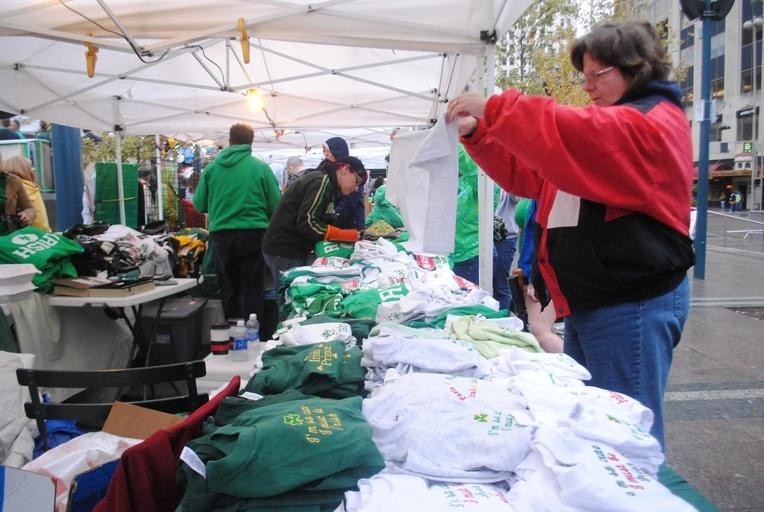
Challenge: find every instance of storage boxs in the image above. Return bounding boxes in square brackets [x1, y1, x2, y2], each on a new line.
[0, 402, 186, 511]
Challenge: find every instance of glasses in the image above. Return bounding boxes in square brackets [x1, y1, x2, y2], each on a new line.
[337, 163, 361, 185]
[583, 65, 615, 81]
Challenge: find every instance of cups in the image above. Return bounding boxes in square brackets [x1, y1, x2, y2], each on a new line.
[210, 316, 245, 354]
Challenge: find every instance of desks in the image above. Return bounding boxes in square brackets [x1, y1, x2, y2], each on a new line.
[47, 275, 199, 400]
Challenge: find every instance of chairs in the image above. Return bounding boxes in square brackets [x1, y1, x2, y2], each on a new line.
[14, 358, 210, 454]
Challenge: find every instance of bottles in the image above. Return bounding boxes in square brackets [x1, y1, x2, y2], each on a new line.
[236, 314, 260, 360]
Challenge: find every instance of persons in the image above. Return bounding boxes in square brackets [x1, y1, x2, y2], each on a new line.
[443, 21, 694, 461]
[140, 168, 208, 231]
[192, 123, 386, 344]
[0, 118, 50, 238]
[728, 192, 736, 213]
[450, 142, 566, 352]
[720, 191, 726, 211]
